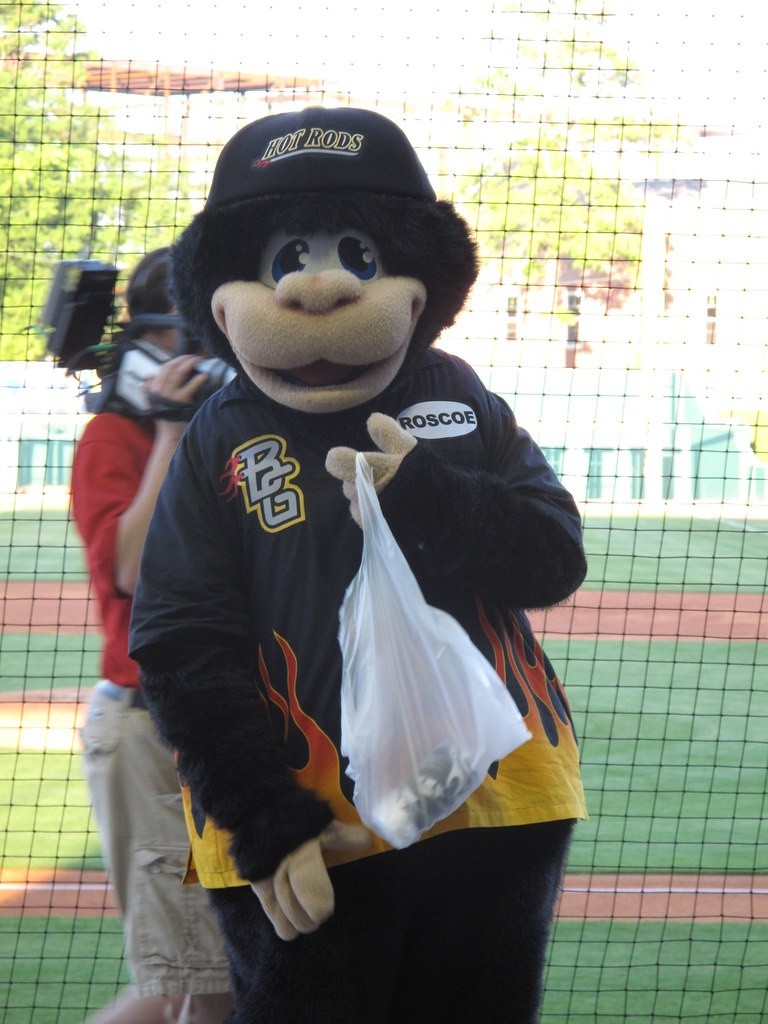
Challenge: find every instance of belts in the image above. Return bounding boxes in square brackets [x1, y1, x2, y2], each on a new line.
[95, 678, 146, 710]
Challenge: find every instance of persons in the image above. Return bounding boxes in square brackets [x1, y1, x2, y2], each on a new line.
[71, 248, 234, 1022]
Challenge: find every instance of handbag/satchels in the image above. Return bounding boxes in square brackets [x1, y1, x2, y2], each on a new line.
[335, 450, 533, 850]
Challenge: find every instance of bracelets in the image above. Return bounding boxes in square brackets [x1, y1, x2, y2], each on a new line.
[147, 393, 193, 423]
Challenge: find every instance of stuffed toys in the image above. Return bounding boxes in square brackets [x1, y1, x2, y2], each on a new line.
[125, 107, 591, 1024]
